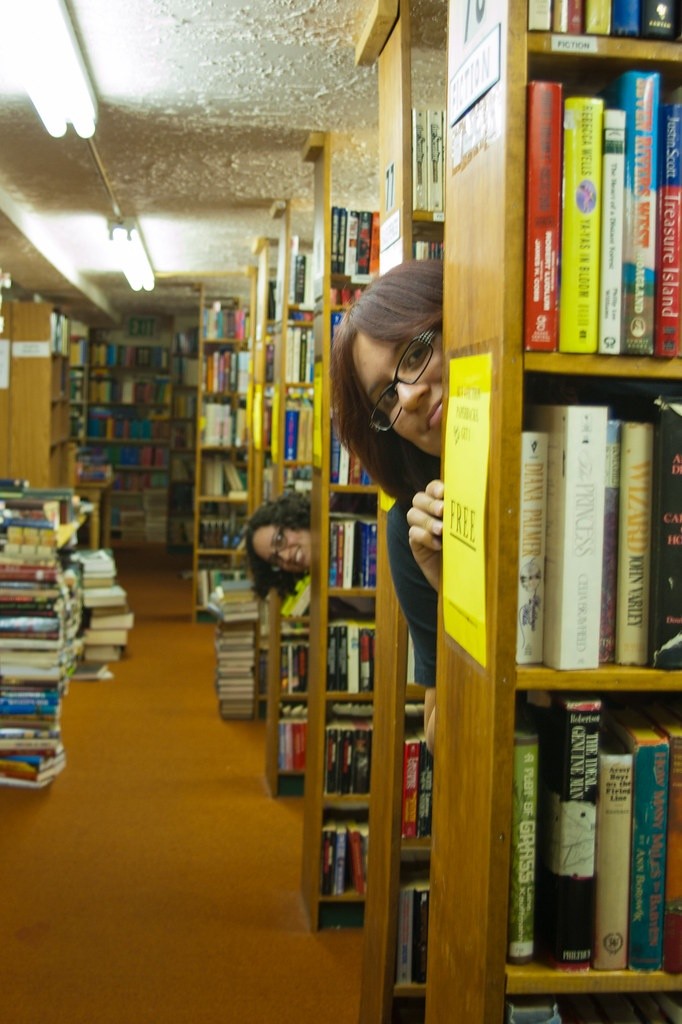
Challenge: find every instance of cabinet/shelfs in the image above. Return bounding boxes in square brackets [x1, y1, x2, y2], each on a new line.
[0, 0, 682, 1024]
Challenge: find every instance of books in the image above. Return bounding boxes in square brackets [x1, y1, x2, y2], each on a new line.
[0, 0, 682, 1024]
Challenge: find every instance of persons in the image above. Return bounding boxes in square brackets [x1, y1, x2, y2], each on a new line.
[328, 255, 444, 753]
[246, 491, 314, 597]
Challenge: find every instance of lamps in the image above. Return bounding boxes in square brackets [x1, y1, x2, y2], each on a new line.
[107, 217, 155, 291]
[0, 0, 99, 139]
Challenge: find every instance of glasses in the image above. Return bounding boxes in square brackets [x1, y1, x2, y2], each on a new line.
[368, 330, 436, 432]
[268, 526, 288, 573]
